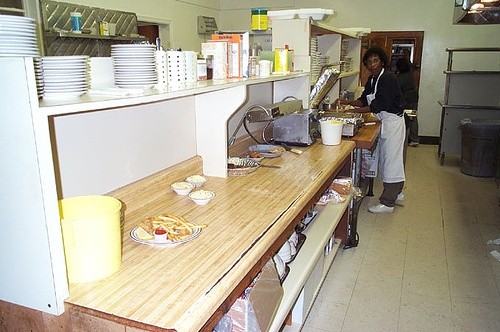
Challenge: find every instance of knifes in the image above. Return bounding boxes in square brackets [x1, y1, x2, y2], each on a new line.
[260, 164, 281, 168]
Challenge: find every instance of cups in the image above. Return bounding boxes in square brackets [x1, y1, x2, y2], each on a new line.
[259, 60, 274, 76]
[69, 12, 82, 34]
[251, 8, 269, 32]
[153, 229, 168, 241]
[108, 23, 117, 35]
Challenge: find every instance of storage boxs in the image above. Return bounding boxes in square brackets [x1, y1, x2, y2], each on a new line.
[226, 258, 284, 332]
[353, 136, 380, 177]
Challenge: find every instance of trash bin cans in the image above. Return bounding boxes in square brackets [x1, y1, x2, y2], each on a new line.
[458, 117, 500, 178]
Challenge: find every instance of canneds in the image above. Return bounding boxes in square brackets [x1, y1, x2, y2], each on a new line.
[250, 8, 268, 31]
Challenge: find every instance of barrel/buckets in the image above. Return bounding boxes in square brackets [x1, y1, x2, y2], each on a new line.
[58, 194, 122, 289]
[318, 117, 346, 146]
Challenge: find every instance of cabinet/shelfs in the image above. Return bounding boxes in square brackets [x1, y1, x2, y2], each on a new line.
[0, 16, 383, 332]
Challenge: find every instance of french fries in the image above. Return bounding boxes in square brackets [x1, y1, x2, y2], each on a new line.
[140, 214, 208, 242]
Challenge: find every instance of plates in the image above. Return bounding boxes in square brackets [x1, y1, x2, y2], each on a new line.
[129, 221, 201, 248]
[239, 152, 264, 161]
[249, 144, 283, 158]
[228, 161, 261, 177]
[309, 37, 329, 81]
[339, 39, 354, 73]
[0, 15, 158, 101]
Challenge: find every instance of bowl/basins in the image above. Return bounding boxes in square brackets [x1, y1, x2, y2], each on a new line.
[184, 175, 208, 188]
[271, 228, 298, 281]
[323, 104, 336, 111]
[170, 181, 195, 196]
[189, 190, 215, 205]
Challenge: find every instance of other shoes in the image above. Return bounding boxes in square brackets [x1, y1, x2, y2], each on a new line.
[368, 203, 395, 214]
[394, 194, 405, 207]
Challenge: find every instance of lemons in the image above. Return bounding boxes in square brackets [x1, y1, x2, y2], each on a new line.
[137, 227, 153, 240]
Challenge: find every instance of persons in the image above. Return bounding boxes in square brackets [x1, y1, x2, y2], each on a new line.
[395, 57, 419, 146]
[338, 46, 411, 213]
